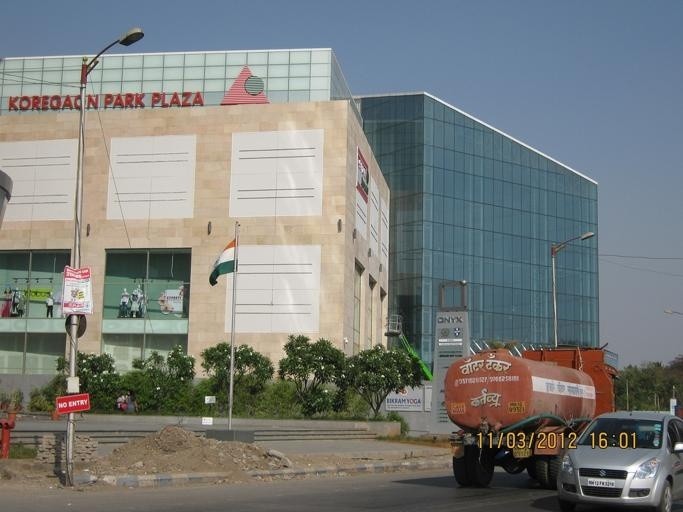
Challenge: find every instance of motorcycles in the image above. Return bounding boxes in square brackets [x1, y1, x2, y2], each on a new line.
[117, 295, 145, 319]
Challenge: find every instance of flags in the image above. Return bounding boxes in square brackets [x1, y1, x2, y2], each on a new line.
[209, 235, 235, 287]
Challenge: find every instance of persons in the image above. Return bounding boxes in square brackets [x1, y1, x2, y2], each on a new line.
[1, 284, 25, 317]
[125, 390, 139, 413]
[45, 291, 55, 318]
[119, 284, 148, 318]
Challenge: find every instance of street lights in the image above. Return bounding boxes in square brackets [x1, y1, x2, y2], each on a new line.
[65, 27, 145, 489]
[550, 231, 595, 348]
[664, 308, 683, 316]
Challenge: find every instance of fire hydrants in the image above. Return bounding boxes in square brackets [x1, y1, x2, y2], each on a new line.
[0, 411, 17, 461]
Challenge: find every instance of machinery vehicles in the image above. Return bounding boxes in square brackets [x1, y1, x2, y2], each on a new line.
[385, 314, 434, 382]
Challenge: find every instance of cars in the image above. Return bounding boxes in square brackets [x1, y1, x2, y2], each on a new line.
[555, 412, 682, 512]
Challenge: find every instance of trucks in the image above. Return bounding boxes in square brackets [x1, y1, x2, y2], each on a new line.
[443, 348, 621, 490]
